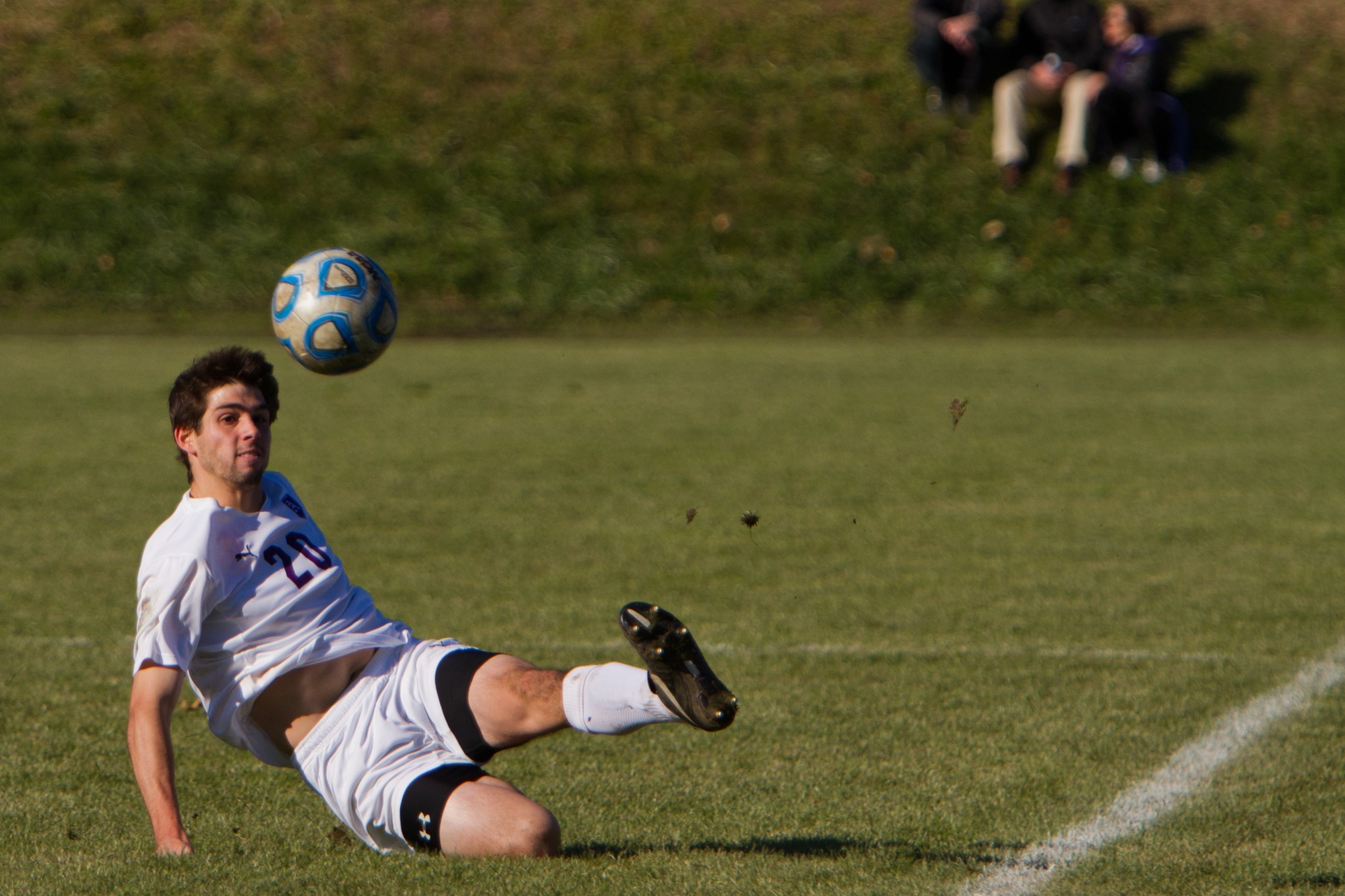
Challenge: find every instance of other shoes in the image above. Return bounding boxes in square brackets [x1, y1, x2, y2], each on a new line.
[1000, 160, 1023, 190]
[619, 602, 738, 731]
[1052, 166, 1072, 191]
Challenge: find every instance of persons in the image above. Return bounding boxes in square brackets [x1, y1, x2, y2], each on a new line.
[123, 347, 735, 865]
[908, 0, 1190, 181]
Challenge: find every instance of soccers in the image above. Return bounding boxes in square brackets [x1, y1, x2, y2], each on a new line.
[271, 246, 398, 377]
